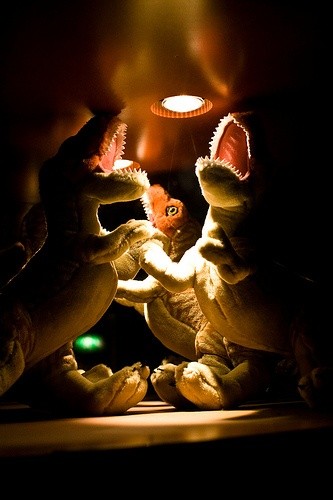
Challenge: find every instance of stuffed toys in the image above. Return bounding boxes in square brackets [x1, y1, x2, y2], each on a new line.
[0, 113, 169, 416]
[138, 111, 332, 410]
[115, 183, 231, 408]
